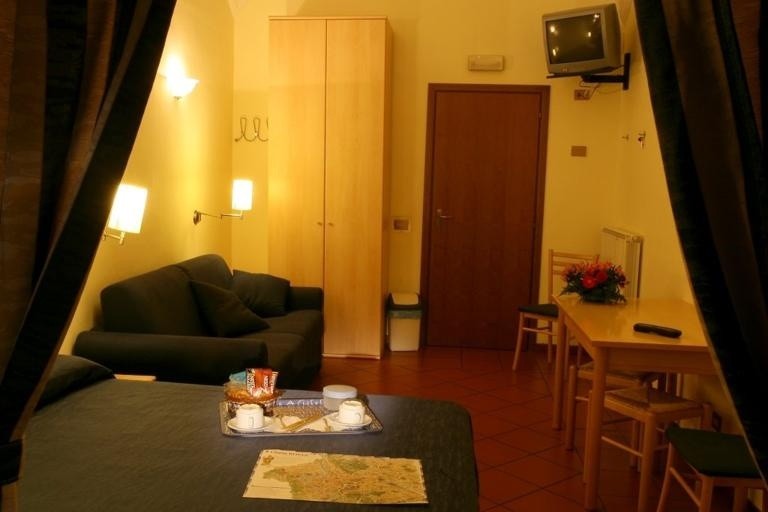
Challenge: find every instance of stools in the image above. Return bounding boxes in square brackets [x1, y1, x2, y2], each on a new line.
[581, 385, 713, 512]
[565, 365, 666, 466]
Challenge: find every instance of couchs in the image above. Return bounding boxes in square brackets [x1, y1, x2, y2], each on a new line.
[72, 252, 324, 390]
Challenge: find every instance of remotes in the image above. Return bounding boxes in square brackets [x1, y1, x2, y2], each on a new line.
[633, 321, 682, 338]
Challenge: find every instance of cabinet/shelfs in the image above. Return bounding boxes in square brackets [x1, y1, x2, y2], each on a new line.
[265, 15, 396, 361]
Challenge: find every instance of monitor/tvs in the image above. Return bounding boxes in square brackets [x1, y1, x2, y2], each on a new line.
[541, 3, 623, 75]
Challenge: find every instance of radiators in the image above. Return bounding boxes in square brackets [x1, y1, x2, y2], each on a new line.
[599, 227, 640, 299]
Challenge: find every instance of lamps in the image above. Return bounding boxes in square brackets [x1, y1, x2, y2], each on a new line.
[165, 73, 198, 101]
[105, 183, 149, 246]
[192, 179, 253, 224]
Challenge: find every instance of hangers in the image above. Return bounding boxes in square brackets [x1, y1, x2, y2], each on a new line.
[234, 116, 268, 142]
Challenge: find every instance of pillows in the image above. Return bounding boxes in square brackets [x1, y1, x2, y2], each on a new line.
[34, 353, 114, 407]
[230, 269, 290, 316]
[190, 280, 270, 338]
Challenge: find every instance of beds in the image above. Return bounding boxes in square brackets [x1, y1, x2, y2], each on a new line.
[14, 378, 479, 512]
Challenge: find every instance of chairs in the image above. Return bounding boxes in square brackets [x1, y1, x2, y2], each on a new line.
[657, 424, 765, 512]
[511, 249, 599, 367]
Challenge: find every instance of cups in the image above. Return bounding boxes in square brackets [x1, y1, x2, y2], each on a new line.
[338, 400, 365, 425]
[236, 404, 264, 429]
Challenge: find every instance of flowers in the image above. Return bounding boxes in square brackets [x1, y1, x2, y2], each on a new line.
[557, 253, 630, 305]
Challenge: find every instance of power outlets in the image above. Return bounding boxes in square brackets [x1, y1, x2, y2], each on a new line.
[636, 131, 645, 144]
[575, 90, 589, 100]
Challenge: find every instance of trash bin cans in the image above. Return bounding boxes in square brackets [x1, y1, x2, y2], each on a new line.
[384, 290, 422, 352]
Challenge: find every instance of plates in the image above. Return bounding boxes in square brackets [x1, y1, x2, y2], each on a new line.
[219, 398, 383, 438]
[329, 414, 372, 428]
[227, 417, 274, 433]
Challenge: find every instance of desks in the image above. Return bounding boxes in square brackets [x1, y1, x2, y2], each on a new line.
[549, 293, 719, 512]
